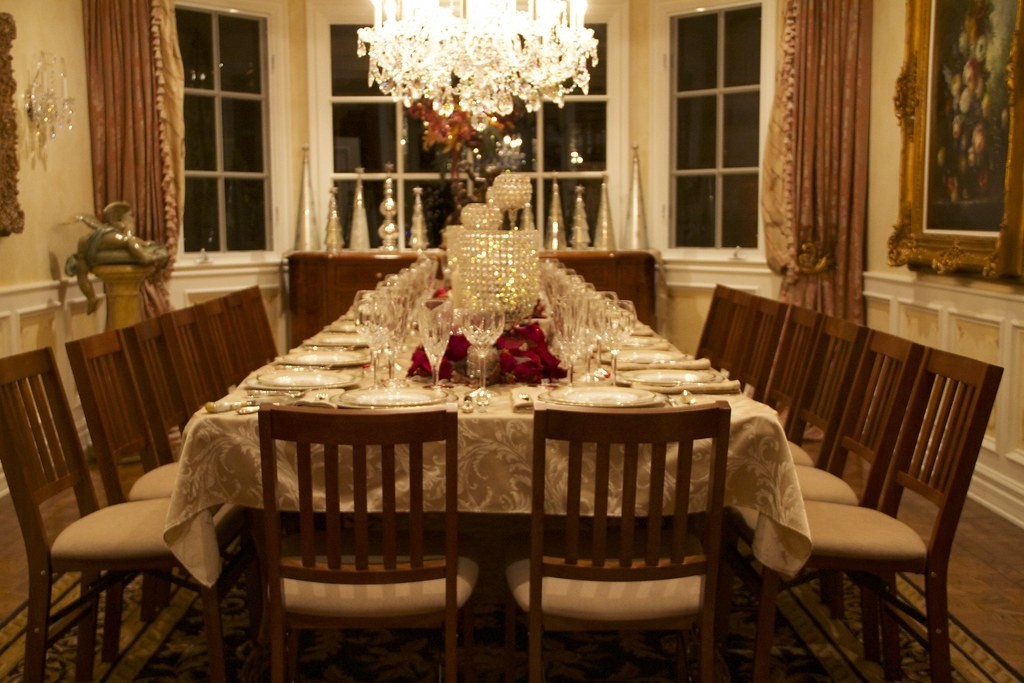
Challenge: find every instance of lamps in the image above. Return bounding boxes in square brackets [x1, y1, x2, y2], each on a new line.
[357, 0, 599, 132]
[21, 53, 75, 139]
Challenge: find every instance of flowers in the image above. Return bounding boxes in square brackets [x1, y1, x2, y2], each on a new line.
[410, 94, 516, 220]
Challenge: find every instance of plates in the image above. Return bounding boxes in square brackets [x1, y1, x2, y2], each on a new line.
[252, 285, 738, 411]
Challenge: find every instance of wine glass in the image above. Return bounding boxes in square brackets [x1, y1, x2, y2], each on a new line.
[351, 251, 636, 399]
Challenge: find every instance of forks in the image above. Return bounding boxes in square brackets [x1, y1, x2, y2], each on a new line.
[315, 385, 329, 402]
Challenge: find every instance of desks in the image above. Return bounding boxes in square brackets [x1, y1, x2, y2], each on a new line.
[161, 291, 813, 683]
[286, 249, 657, 352]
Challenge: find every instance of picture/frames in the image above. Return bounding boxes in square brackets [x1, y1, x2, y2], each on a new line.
[888, 0, 1024, 281]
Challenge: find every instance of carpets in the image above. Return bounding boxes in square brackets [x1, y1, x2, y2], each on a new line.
[1, 538, 1024, 683]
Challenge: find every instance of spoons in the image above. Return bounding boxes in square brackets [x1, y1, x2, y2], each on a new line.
[680, 389, 693, 407]
[477, 398, 488, 412]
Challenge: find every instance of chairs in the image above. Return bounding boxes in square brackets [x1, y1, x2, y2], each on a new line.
[1, 283, 346, 683]
[502, 401, 730, 683]
[256, 401, 480, 683]
[695, 281, 1005, 683]
